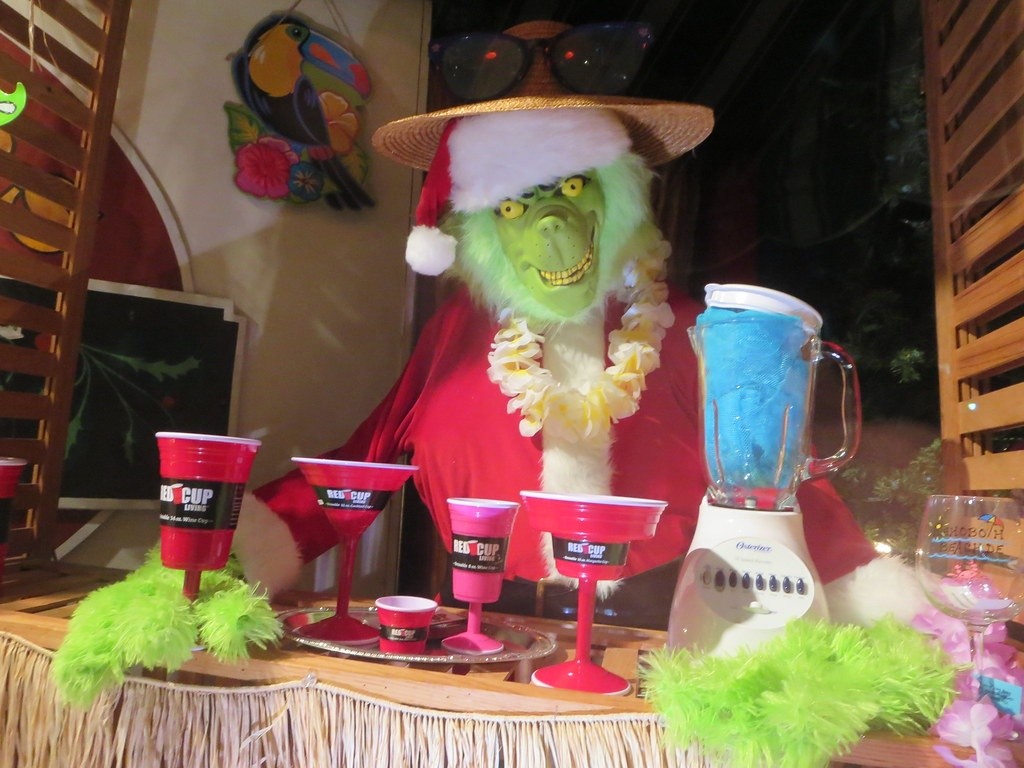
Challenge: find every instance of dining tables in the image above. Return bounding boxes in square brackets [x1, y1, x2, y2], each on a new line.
[0, 552, 946, 768]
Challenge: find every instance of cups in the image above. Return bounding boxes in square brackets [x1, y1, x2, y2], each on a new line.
[0, 458, 28, 582]
[374, 595, 438, 655]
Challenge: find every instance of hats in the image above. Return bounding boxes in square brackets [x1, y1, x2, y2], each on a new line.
[371, 22, 713, 171]
[404, 110, 633, 276]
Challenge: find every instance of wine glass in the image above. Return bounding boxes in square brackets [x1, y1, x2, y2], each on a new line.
[520, 490, 668, 695]
[290, 457, 419, 644]
[155, 431, 262, 651]
[916, 494, 1024, 701]
[441, 496, 520, 658]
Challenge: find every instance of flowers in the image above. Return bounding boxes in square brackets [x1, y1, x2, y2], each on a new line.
[225, 89, 372, 213]
[910, 604, 1024, 767]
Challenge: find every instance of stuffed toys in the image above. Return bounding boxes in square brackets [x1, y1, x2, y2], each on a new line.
[54, 20, 970, 765]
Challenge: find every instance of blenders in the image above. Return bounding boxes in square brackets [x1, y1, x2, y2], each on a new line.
[667, 283, 861, 654]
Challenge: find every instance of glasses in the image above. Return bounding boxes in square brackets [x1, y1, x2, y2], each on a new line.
[430, 17, 660, 99]
